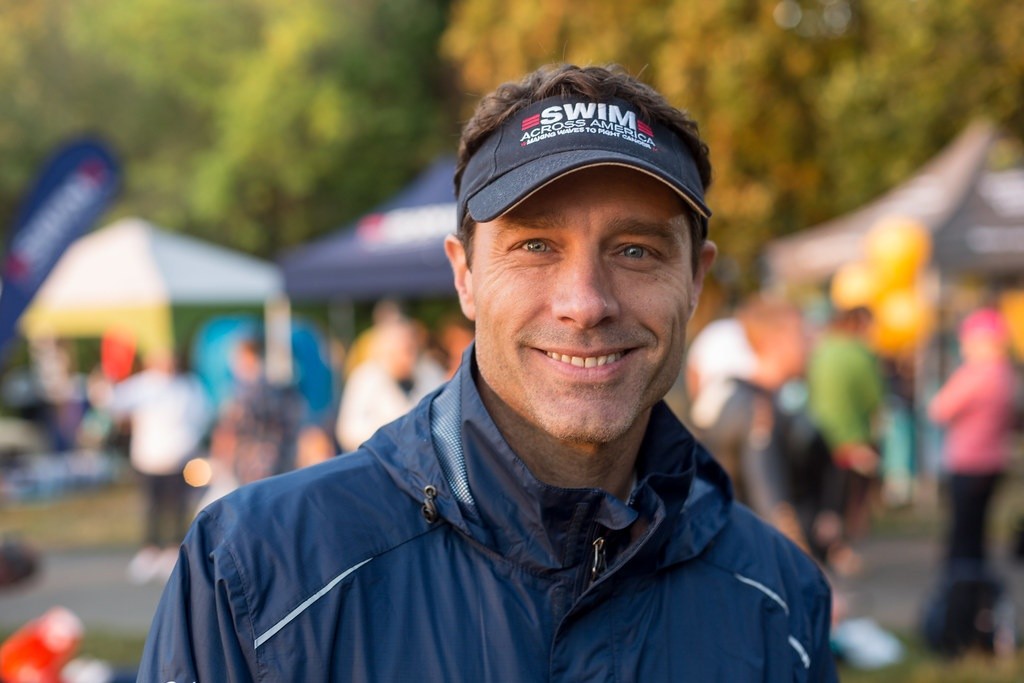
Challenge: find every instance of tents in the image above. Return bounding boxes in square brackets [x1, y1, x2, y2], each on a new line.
[274, 148, 457, 303]
[27, 215, 289, 357]
[760, 119, 1023, 279]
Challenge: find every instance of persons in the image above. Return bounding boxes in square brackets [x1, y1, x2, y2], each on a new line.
[20, 278, 1022, 661]
[134, 33, 837, 682]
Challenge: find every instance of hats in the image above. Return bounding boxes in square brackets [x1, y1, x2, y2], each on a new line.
[457, 94, 711, 227]
